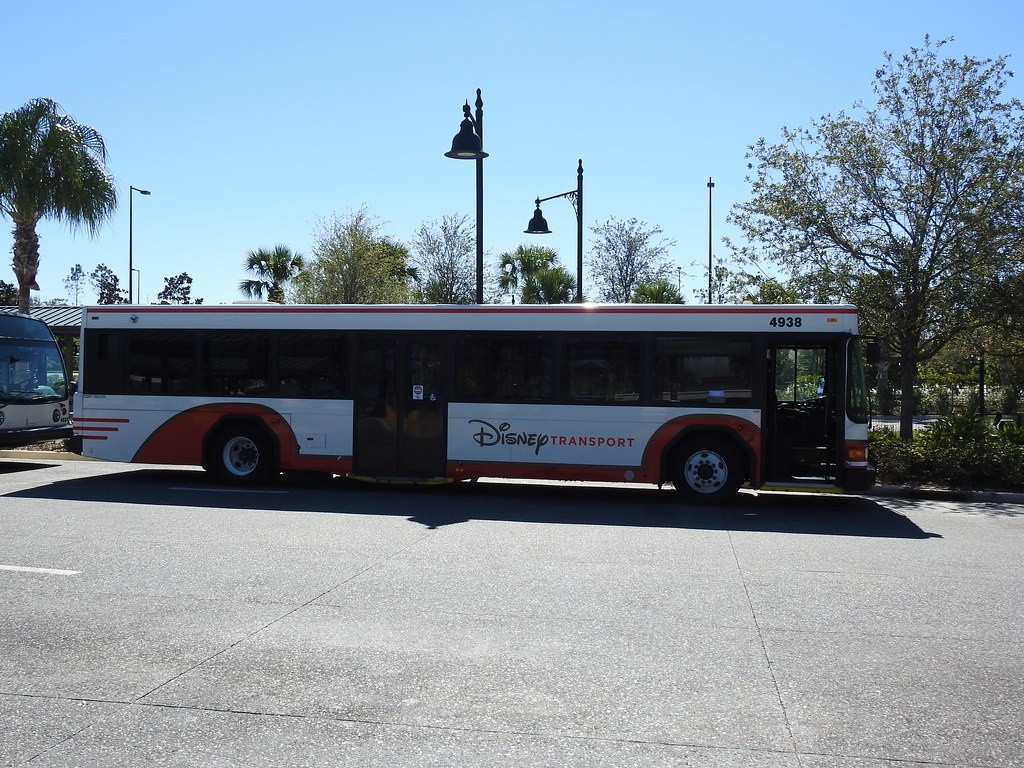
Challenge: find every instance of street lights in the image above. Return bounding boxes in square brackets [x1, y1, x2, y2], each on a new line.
[703, 175, 716, 305]
[443, 90, 488, 303]
[969, 305, 991, 452]
[128, 182, 150, 305]
[524, 159, 585, 298]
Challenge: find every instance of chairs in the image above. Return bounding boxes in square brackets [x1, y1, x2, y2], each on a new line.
[14, 361, 30, 388]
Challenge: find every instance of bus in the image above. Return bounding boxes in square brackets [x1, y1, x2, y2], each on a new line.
[0, 308, 69, 430]
[62, 301, 881, 504]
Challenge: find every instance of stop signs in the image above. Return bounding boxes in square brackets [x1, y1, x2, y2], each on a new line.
[414, 386, 422, 394]
